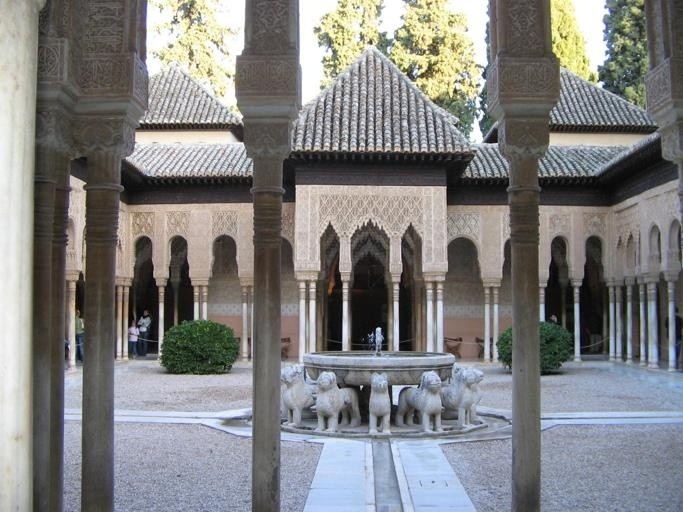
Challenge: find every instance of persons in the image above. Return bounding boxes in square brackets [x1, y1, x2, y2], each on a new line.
[665, 306, 682, 370]
[549, 314, 558, 324]
[126, 319, 138, 360]
[579, 311, 589, 353]
[588, 308, 603, 351]
[136, 309, 152, 357]
[74, 308, 85, 364]
